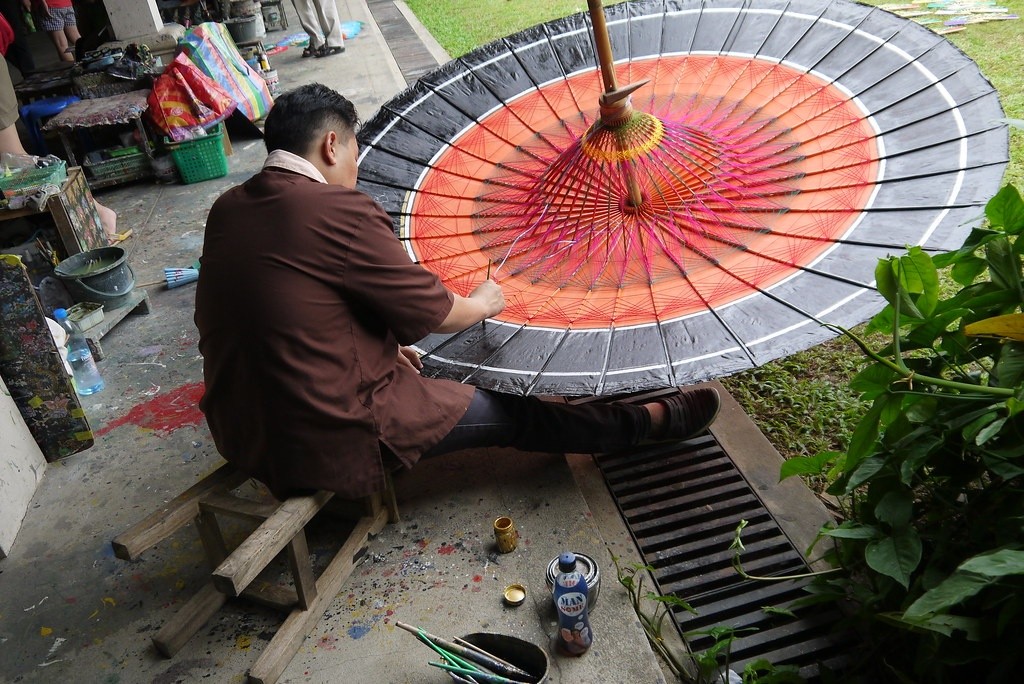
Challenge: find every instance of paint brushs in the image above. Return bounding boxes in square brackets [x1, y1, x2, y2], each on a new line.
[481, 257, 492, 327]
[395, 620, 540, 684]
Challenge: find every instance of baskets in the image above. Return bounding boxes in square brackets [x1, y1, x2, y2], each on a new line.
[0, 159, 68, 199]
[83, 148, 149, 182]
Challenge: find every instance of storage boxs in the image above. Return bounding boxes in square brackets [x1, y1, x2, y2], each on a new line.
[0, 158, 66, 197]
[65, 301, 104, 332]
[161, 123, 228, 184]
[82, 144, 145, 179]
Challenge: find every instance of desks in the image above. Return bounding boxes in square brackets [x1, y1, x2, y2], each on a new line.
[0, 166, 109, 256]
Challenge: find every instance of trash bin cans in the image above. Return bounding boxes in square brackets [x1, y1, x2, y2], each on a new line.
[224, 14, 257, 44]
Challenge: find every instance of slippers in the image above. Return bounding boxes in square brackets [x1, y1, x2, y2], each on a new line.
[107, 227, 133, 247]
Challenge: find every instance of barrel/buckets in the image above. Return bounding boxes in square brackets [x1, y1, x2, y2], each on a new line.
[231, 1, 266, 38]
[223, 15, 258, 43]
[262, 69, 280, 96]
[54, 246, 136, 312]
[444, 632, 550, 684]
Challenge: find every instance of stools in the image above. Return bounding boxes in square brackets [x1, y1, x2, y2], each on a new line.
[18, 94, 81, 159]
[110, 445, 402, 683]
[42, 87, 156, 190]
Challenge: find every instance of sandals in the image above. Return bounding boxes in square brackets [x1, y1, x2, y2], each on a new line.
[302, 45, 317, 57]
[314, 45, 346, 57]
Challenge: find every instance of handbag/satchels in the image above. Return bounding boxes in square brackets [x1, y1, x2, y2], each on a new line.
[142, 51, 239, 144]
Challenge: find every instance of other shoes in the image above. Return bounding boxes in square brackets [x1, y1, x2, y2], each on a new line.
[639, 386, 721, 446]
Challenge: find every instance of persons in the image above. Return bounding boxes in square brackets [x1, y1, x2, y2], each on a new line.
[0, 13, 116, 245]
[193, 81, 722, 499]
[0, 0, 36, 77]
[40, 0, 87, 63]
[291, 0, 346, 58]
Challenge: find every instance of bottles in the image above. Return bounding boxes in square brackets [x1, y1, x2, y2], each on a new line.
[24, 250, 39, 277]
[551, 552, 593, 656]
[54, 308, 105, 396]
[23, 12, 36, 33]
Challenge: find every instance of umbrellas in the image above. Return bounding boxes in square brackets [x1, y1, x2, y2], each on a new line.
[134, 266, 200, 293]
[329, 0, 1010, 399]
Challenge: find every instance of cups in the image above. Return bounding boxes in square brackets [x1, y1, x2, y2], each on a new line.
[545, 552, 601, 614]
[494, 517, 516, 554]
[152, 152, 179, 184]
